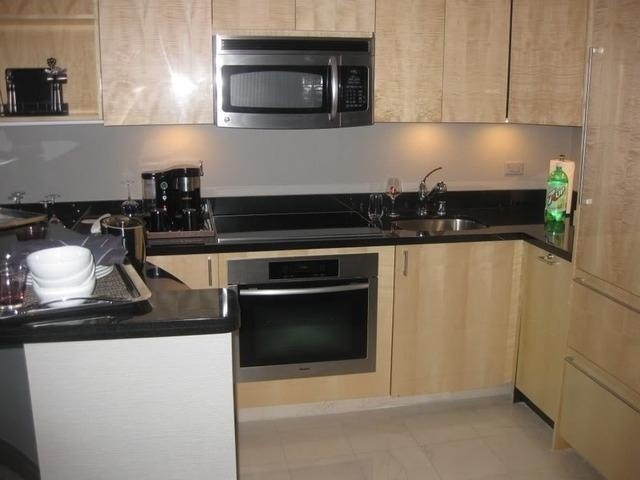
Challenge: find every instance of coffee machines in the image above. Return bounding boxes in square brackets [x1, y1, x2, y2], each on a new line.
[156, 164, 204, 229]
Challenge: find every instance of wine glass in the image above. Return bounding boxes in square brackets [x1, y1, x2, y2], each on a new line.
[120, 178, 139, 215]
[386, 177, 402, 219]
[8, 190, 62, 237]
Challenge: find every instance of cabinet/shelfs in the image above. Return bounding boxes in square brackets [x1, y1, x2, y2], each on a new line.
[550, 275, 640, 480]
[372, 0, 511, 124]
[0, 0, 101, 126]
[100, 0, 215, 127]
[149, 254, 220, 289]
[214, 0, 376, 34]
[568, 0, 640, 308]
[390, 240, 521, 406]
[514, 240, 572, 430]
[510, 0, 593, 127]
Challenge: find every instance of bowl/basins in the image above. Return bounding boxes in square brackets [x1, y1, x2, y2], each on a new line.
[26, 245, 97, 306]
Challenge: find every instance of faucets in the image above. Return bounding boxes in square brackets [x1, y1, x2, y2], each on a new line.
[419, 181, 447, 201]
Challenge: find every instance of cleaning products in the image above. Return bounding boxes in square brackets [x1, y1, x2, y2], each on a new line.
[543, 164, 568, 231]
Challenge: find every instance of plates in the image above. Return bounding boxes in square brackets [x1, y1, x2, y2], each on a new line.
[25, 263, 115, 285]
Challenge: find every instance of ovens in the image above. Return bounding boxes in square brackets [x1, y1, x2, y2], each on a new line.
[226, 253, 381, 384]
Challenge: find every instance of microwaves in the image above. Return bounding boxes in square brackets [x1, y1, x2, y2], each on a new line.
[218, 39, 374, 130]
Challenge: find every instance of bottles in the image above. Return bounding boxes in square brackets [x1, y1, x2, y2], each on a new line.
[545, 153, 575, 234]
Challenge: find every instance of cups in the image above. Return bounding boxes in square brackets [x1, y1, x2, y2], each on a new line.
[368, 193, 384, 222]
[0, 260, 25, 318]
[99, 216, 148, 273]
[141, 171, 157, 216]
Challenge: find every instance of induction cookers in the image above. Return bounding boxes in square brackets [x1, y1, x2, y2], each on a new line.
[212, 211, 375, 242]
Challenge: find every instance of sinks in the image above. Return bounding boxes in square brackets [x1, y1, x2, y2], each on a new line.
[392, 216, 487, 232]
